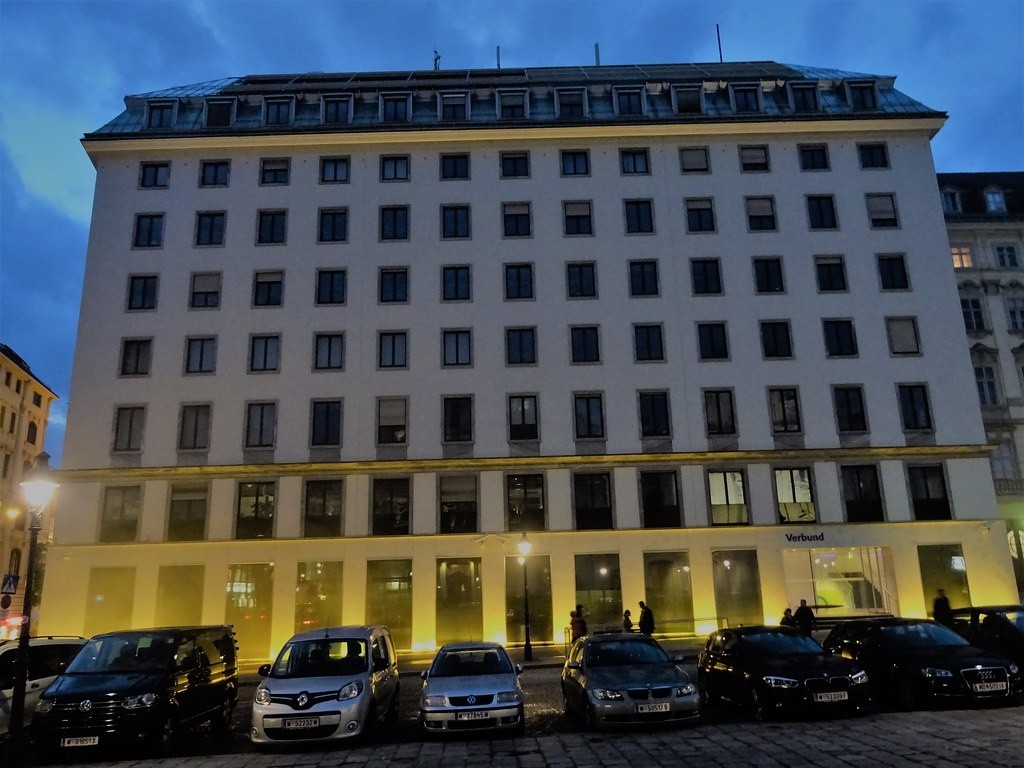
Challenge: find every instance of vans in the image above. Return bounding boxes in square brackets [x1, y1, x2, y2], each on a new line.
[31, 625, 241, 761]
[248, 624, 402, 745]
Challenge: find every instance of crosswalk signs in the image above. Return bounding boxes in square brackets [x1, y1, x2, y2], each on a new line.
[1, 574, 21, 596]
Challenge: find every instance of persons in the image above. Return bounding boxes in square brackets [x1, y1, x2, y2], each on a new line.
[933, 589, 954, 631]
[780, 600, 816, 638]
[623, 610, 633, 633]
[570, 604, 590, 644]
[639, 601, 655, 636]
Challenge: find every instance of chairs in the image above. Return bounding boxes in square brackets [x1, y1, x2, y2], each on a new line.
[110, 644, 135, 671]
[483, 652, 498, 665]
[136, 647, 153, 662]
[446, 654, 461, 666]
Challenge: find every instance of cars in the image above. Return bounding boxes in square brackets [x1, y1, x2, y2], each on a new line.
[698, 625, 871, 725]
[0, 636, 99, 743]
[824, 617, 1024, 710]
[562, 634, 701, 729]
[943, 604, 1024, 658]
[416, 643, 529, 736]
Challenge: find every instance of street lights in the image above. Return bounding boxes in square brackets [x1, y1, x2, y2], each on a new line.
[6, 451, 61, 768]
[516, 530, 534, 661]
